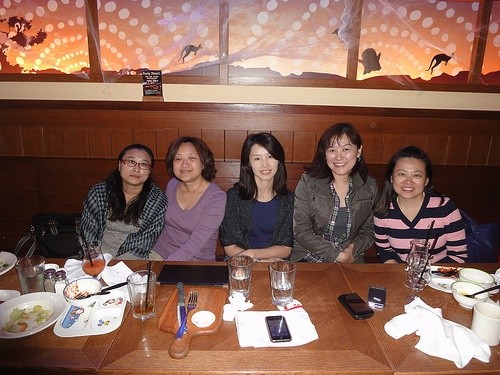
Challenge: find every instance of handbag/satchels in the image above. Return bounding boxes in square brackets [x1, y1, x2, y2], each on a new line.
[12, 212, 83, 260]
[456, 207, 500, 263]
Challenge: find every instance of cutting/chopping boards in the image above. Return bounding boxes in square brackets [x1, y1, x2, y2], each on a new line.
[156, 284, 227, 359]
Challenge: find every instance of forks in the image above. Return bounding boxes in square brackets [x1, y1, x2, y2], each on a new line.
[174, 291, 199, 340]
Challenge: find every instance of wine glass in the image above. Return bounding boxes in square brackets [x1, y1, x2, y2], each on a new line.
[404, 239, 431, 288]
[405, 251, 432, 301]
[81, 240, 106, 280]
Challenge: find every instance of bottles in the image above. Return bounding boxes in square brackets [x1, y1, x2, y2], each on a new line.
[42, 267, 57, 293]
[54, 270, 68, 296]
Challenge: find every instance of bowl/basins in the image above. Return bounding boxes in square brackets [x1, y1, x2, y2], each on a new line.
[451, 280, 490, 309]
[63, 277, 102, 307]
[458, 268, 494, 290]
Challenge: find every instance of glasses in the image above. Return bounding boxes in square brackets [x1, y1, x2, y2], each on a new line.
[119, 157, 153, 170]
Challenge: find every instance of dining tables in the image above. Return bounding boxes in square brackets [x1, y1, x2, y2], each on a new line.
[99, 261, 395, 375]
[0, 260, 151, 375]
[339, 263, 500, 375]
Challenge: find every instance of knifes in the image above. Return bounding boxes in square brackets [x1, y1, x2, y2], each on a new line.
[177, 281, 188, 334]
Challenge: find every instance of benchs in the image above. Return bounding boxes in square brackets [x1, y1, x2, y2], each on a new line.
[0, 156, 500, 263]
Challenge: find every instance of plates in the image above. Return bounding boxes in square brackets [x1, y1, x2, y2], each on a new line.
[0, 251, 17, 276]
[428, 266, 499, 295]
[0, 289, 20, 305]
[0, 291, 66, 339]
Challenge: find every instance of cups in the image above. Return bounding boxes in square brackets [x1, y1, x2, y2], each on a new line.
[227, 255, 254, 296]
[268, 260, 296, 305]
[15, 255, 46, 295]
[127, 269, 156, 319]
[471, 302, 500, 347]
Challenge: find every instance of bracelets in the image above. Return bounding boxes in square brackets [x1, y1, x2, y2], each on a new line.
[255, 258, 262, 262]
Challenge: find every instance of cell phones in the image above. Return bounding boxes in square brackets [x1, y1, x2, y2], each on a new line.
[368, 286, 386, 310]
[338, 292, 375, 320]
[265, 316, 292, 343]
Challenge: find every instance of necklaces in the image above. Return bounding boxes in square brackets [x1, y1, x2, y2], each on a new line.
[258, 191, 273, 198]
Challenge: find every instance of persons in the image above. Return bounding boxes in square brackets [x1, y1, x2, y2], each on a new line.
[219, 133, 295, 262]
[372, 146, 467, 264]
[149, 138, 228, 262]
[74, 143, 167, 260]
[288, 122, 382, 263]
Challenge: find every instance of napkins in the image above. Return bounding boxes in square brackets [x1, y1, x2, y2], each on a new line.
[55, 253, 145, 301]
[235, 306, 319, 348]
[383, 296, 491, 369]
